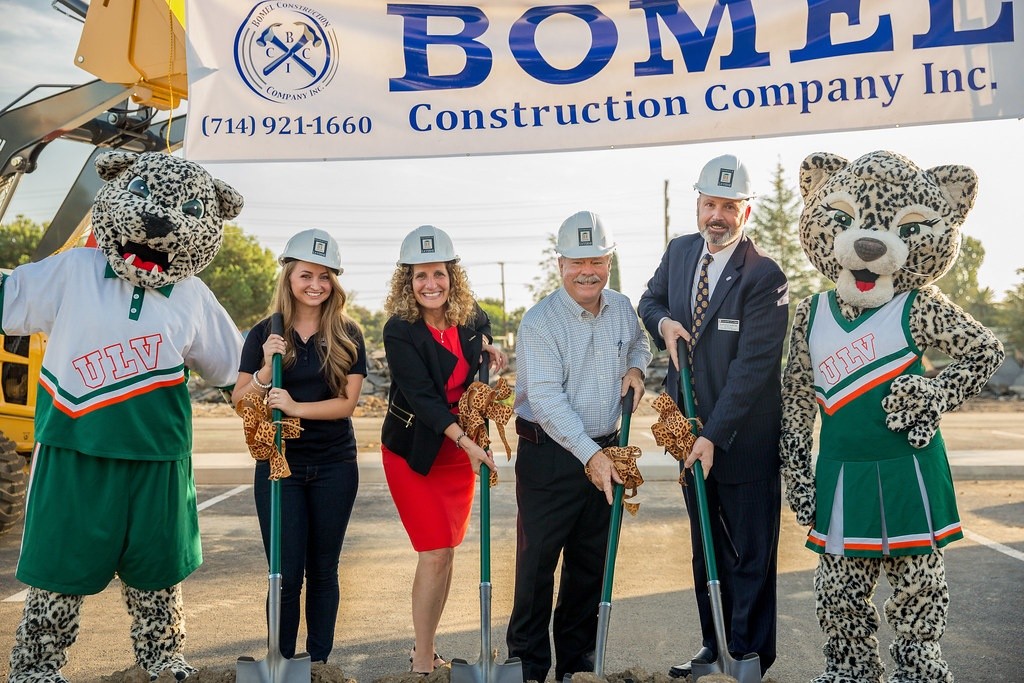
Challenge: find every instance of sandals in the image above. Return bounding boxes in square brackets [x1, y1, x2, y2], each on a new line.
[408, 646, 444, 677]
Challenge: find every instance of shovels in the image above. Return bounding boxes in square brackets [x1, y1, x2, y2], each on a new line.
[233, 312, 313, 683]
[449, 349, 524, 683]
[562, 384, 635, 683]
[676, 335, 764, 683]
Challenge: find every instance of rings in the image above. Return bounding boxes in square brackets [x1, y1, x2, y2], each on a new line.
[274, 393, 278, 400]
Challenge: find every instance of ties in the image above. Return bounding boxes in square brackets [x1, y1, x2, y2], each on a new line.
[686, 254, 714, 372]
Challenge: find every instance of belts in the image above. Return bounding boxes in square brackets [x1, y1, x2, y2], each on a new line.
[544, 432, 618, 448]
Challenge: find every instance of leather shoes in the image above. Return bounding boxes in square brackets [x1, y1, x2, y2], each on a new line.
[668, 647, 713, 677]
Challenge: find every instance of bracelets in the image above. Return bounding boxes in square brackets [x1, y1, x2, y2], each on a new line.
[250, 378, 270, 392]
[253, 370, 272, 388]
[456, 432, 469, 450]
[482, 337, 489, 344]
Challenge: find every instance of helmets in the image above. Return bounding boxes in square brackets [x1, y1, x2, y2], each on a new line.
[693, 153, 755, 200]
[398, 226, 460, 265]
[279, 228, 343, 276]
[555, 211, 617, 259]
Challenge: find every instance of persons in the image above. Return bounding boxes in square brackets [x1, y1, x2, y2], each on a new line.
[378, 224, 506, 678]
[636, 154, 788, 680]
[507, 211, 652, 683]
[232, 227, 367, 666]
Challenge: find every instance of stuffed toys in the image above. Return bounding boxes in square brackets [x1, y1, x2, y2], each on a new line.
[778, 149, 1007, 683]
[0, 148, 247, 683]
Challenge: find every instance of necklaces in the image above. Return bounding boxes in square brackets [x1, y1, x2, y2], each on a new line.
[292, 322, 319, 341]
[422, 314, 449, 344]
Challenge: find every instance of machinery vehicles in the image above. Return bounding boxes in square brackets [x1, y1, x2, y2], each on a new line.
[0, 0, 191, 536]
